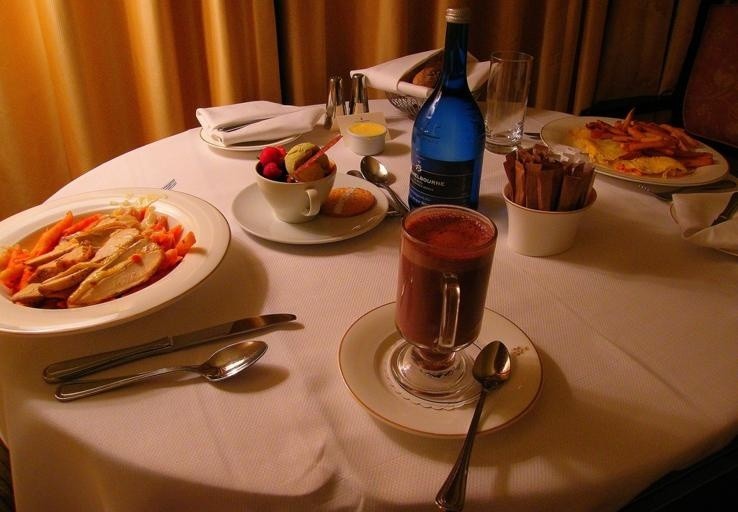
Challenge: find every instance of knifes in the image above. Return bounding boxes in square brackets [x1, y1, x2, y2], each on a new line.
[708, 192, 737, 227]
[41, 312, 297, 385]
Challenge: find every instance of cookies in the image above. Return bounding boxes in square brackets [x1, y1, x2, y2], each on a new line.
[323, 187, 375, 217]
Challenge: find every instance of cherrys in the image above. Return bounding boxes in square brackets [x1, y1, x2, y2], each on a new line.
[257, 145, 286, 177]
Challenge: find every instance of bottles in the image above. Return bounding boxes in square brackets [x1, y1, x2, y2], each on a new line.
[348, 72, 370, 115]
[321, 75, 348, 132]
[407, 5, 486, 212]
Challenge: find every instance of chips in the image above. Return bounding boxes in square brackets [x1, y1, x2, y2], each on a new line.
[563, 126, 686, 177]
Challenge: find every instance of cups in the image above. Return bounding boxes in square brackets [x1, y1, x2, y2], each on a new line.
[346, 120, 388, 156]
[252, 159, 338, 224]
[501, 182, 598, 258]
[483, 50, 534, 156]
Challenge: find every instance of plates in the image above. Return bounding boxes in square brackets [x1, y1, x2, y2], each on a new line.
[0, 185, 232, 339]
[337, 301, 546, 442]
[540, 116, 731, 188]
[199, 127, 302, 153]
[231, 172, 390, 246]
[669, 201, 738, 259]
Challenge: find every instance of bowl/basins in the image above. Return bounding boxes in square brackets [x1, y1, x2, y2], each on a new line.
[384, 90, 482, 124]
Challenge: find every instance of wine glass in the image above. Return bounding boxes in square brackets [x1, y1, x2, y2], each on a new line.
[388, 203, 499, 404]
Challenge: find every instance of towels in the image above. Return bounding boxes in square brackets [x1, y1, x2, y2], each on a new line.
[349, 47, 500, 98]
[670, 174, 738, 251]
[194, 100, 327, 146]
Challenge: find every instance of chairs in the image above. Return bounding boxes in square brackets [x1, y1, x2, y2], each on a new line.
[578, 0, 738, 175]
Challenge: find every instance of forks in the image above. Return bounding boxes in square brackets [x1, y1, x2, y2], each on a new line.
[638, 179, 737, 203]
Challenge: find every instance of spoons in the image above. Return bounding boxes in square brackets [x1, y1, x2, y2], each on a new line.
[360, 155, 410, 219]
[433, 339, 511, 512]
[52, 338, 269, 403]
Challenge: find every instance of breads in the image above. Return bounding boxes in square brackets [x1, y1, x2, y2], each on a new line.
[406, 55, 443, 87]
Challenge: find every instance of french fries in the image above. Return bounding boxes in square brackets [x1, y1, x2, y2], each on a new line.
[588, 103, 714, 168]
